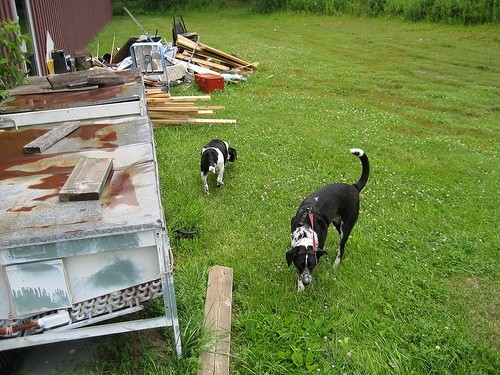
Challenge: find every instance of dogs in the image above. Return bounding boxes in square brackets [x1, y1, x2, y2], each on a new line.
[200, 139, 238, 196]
[284, 148, 370, 292]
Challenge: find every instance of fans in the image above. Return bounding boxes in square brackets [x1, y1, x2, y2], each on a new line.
[130, 42, 164, 75]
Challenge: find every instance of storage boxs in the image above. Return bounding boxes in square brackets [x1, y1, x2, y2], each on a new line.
[195, 73, 224, 93]
